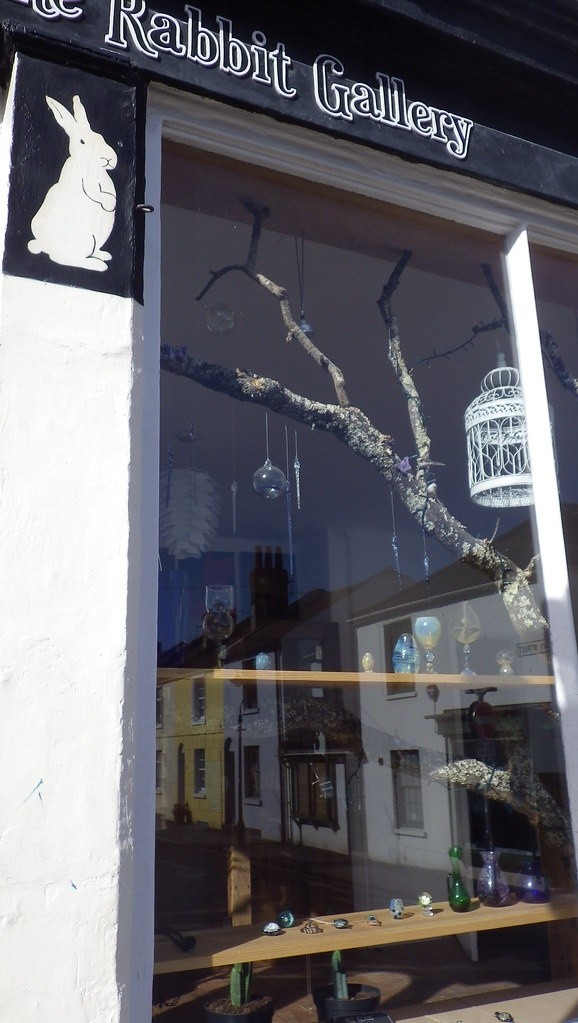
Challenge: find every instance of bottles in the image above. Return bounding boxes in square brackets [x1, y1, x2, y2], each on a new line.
[476, 851, 511, 908]
[515, 861, 551, 904]
[446, 846, 471, 912]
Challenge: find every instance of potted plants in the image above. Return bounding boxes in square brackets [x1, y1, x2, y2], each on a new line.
[311, 949, 383, 1023]
[200, 962, 275, 1023]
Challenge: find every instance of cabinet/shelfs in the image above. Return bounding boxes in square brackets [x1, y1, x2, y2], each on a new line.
[154, 665, 578, 976]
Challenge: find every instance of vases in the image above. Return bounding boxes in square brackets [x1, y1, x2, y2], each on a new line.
[516, 860, 552, 905]
[476, 850, 511, 908]
[445, 846, 471, 913]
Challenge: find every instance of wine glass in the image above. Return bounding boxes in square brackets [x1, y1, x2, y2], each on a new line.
[414, 616, 441, 675]
[205, 585, 234, 669]
